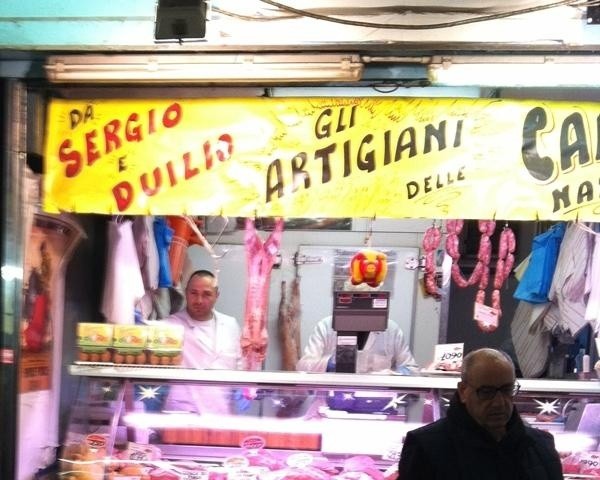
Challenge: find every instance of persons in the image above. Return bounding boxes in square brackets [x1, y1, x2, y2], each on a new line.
[397, 346, 562, 479]
[296, 277, 415, 418]
[36, 240, 56, 350]
[159, 270, 242, 412]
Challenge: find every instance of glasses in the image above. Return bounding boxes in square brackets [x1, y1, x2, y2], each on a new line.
[466, 380, 520, 400]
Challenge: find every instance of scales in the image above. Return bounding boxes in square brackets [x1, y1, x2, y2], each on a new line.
[327, 291, 396, 414]
